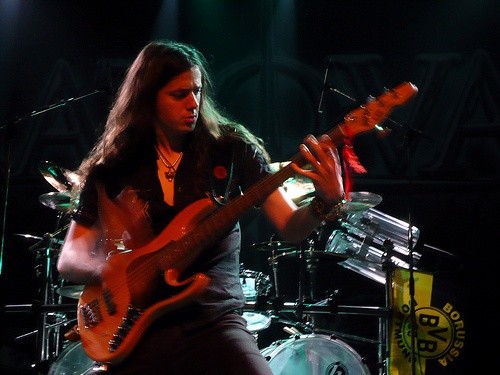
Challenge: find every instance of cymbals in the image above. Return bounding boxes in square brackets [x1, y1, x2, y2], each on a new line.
[267, 248, 350, 266]
[39, 191, 72, 210]
[40, 164, 79, 193]
[56, 283, 85, 299]
[333, 191, 383, 212]
[241, 161, 317, 208]
[249, 241, 298, 251]
[15, 233, 64, 244]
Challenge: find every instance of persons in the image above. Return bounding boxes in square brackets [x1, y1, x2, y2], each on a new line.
[55, 38, 346, 375]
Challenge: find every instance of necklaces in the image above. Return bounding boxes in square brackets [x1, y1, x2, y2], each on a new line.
[151, 139, 185, 181]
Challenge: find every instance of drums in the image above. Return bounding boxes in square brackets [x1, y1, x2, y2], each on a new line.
[237, 269, 271, 310]
[48, 339, 95, 375]
[259, 334, 372, 375]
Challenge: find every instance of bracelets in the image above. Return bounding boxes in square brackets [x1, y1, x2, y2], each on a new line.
[311, 196, 343, 222]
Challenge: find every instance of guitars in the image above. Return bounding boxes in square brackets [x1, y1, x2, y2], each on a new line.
[77, 81, 418, 363]
